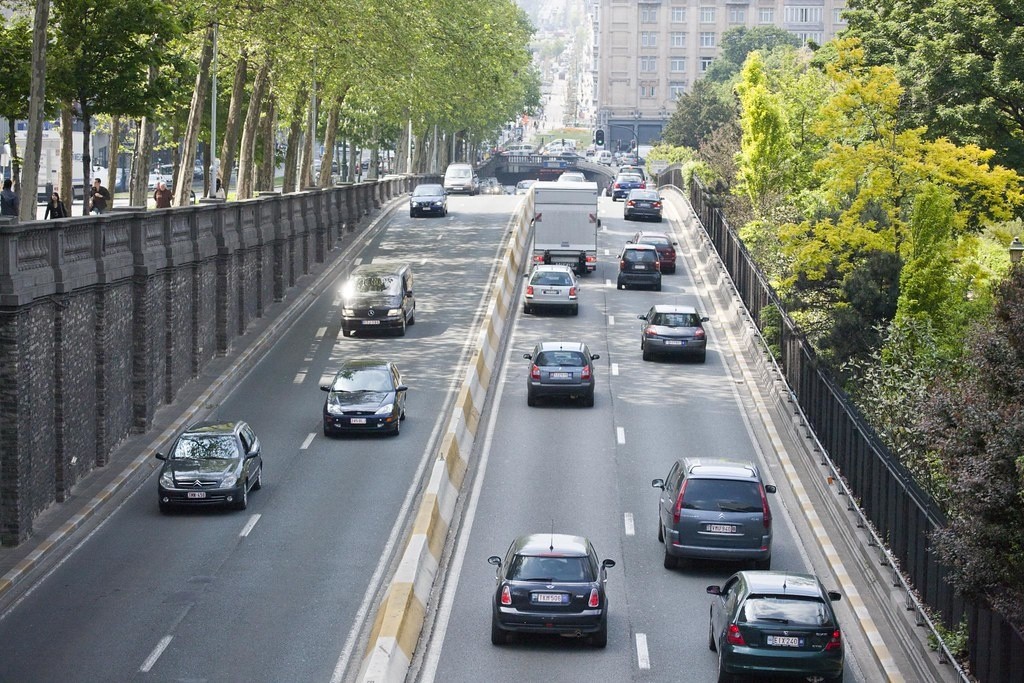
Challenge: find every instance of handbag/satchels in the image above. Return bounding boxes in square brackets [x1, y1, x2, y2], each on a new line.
[88, 195, 95, 211]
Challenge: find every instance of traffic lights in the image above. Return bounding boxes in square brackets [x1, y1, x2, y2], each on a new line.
[632, 141, 636, 149]
[596, 129, 604, 146]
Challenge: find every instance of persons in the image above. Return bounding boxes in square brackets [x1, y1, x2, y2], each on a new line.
[0, 179, 20, 216]
[44, 192, 68, 220]
[153, 182, 174, 208]
[207, 178, 225, 201]
[88, 179, 110, 215]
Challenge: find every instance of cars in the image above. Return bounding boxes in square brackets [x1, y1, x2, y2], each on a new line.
[320, 360, 408, 439]
[637, 304, 709, 365]
[624, 188, 665, 223]
[147, 142, 396, 189]
[441, 162, 537, 196]
[523, 341, 600, 408]
[653, 456, 777, 572]
[557, 148, 646, 202]
[408, 183, 450, 218]
[706, 570, 845, 683]
[156, 419, 263, 515]
[616, 244, 662, 291]
[487, 533, 615, 650]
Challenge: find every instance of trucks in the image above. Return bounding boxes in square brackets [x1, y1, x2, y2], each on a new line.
[534, 179, 601, 275]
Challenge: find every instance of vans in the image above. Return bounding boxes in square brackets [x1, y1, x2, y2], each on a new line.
[504, 139, 576, 156]
[338, 262, 416, 337]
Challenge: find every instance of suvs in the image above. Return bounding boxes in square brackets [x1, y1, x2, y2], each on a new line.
[626, 231, 678, 274]
[522, 263, 579, 316]
